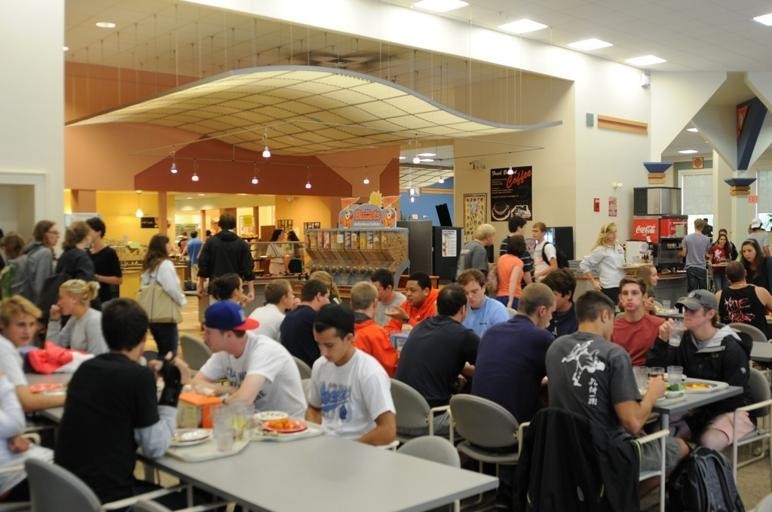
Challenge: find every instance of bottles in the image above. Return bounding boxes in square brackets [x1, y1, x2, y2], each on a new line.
[646, 233, 658, 259]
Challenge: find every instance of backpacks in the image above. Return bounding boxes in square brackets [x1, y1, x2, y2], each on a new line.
[542, 242, 569, 268]
[668, 446, 745, 512]
[0, 246, 42, 302]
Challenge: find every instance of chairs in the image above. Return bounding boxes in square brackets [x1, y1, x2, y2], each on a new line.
[2, 432, 41, 512]
[388, 377, 465, 446]
[731, 370, 772, 485]
[1, 320, 772, 404]
[23, 457, 190, 511]
[448, 393, 531, 504]
[397, 435, 463, 511]
[518, 421, 670, 512]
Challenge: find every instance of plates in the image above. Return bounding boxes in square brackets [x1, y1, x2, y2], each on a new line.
[167, 429, 210, 447]
[253, 411, 307, 433]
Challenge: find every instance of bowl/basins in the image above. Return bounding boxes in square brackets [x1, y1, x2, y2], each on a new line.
[568, 260, 581, 268]
[665, 391, 685, 399]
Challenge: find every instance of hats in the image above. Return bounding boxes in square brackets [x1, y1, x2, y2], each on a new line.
[312, 304, 354, 332]
[751, 217, 762, 229]
[674, 289, 717, 311]
[205, 301, 259, 331]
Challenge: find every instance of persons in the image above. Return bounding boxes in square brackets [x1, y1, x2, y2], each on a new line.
[454, 214, 557, 310]
[267, 229, 303, 276]
[0, 261, 772, 512]
[679, 216, 772, 296]
[178, 229, 213, 288]
[196, 212, 255, 306]
[579, 222, 627, 305]
[1, 217, 122, 312]
[140, 235, 186, 363]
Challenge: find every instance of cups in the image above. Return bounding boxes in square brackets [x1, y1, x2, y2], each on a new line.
[212, 403, 233, 452]
[669, 320, 685, 347]
[663, 299, 671, 311]
[633, 365, 682, 390]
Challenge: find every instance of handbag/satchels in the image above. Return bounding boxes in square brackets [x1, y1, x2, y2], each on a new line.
[135, 279, 182, 324]
[36, 272, 76, 323]
